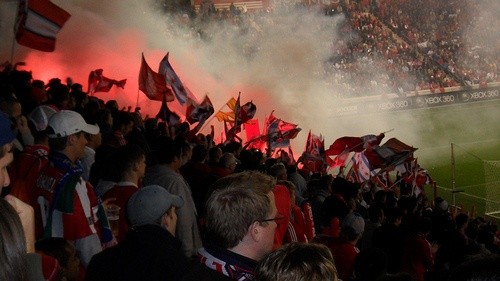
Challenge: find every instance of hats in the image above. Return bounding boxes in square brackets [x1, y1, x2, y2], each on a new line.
[28, 105, 61, 131]
[341, 214, 364, 234]
[0, 111, 17, 146]
[126, 185, 184, 224]
[47, 110, 100, 138]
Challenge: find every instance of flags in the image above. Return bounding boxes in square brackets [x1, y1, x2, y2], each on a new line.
[14, 0, 72, 54]
[88, 68, 127, 95]
[159, 54, 188, 105]
[138, 52, 170, 102]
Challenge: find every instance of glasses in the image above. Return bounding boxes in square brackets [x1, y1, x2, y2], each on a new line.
[248, 214, 285, 231]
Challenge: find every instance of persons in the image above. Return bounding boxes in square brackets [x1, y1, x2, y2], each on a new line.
[0, 0, 500, 281]
